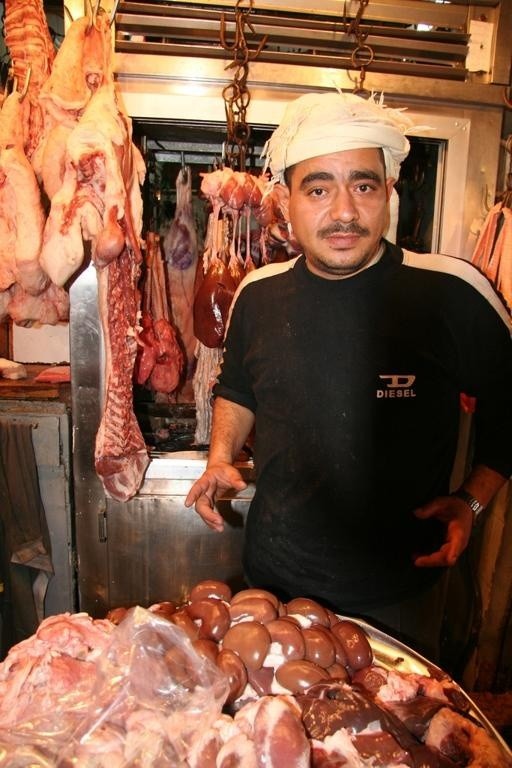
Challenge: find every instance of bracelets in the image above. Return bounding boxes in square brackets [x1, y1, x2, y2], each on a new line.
[452, 486, 485, 525]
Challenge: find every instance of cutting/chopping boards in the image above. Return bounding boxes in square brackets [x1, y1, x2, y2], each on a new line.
[1, 359, 71, 398]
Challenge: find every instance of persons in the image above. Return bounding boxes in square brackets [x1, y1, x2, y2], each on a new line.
[183, 89, 511, 665]
